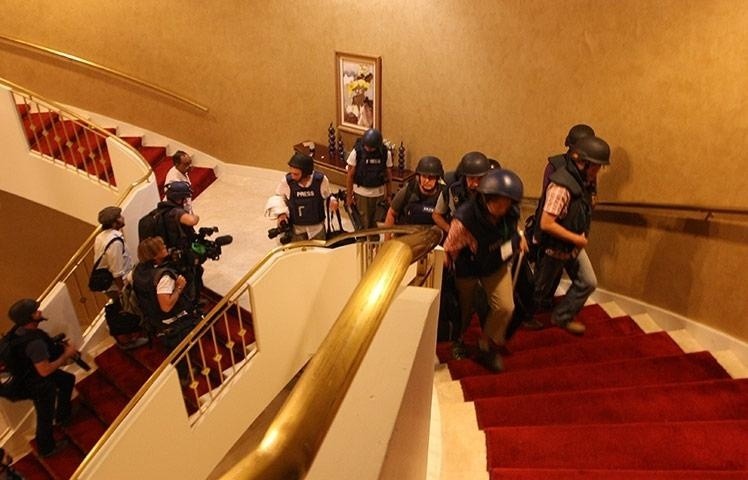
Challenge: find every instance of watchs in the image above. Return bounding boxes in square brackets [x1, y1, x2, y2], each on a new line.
[176, 285, 183, 291]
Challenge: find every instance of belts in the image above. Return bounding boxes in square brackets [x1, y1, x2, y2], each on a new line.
[545, 247, 579, 260]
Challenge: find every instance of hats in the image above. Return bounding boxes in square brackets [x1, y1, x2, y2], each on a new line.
[98, 206, 122, 225]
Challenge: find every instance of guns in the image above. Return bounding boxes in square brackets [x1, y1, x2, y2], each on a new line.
[337, 189, 364, 231]
[268, 224, 292, 239]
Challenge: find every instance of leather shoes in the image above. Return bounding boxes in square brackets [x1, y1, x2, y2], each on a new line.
[119, 338, 149, 351]
[127, 333, 140, 340]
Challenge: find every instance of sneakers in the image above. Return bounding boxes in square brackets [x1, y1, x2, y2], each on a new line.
[41, 436, 67, 459]
[478, 351, 502, 372]
[453, 340, 465, 359]
[181, 367, 197, 387]
[551, 315, 586, 334]
[534, 312, 547, 328]
[57, 408, 81, 427]
[497, 344, 511, 354]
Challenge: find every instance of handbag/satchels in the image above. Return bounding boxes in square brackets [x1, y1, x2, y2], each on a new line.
[153, 269, 202, 354]
[437, 252, 462, 343]
[324, 194, 356, 249]
[120, 281, 145, 326]
[104, 297, 145, 336]
[89, 237, 125, 291]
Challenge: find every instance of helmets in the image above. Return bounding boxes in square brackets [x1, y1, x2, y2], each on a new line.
[364, 129, 383, 147]
[565, 124, 596, 146]
[9, 298, 41, 323]
[417, 156, 444, 176]
[488, 159, 500, 169]
[480, 170, 524, 201]
[288, 154, 314, 177]
[461, 151, 491, 176]
[166, 180, 193, 199]
[573, 137, 611, 165]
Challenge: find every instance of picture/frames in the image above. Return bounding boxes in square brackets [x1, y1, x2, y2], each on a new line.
[333, 50, 382, 137]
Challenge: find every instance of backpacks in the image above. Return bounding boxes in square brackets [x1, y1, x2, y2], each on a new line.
[0, 324, 48, 401]
[139, 205, 182, 249]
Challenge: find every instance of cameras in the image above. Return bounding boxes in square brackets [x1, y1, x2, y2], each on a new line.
[377, 199, 389, 209]
[267, 221, 294, 245]
[189, 227, 233, 262]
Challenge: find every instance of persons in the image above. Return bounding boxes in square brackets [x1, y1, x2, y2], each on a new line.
[385, 157, 448, 342]
[0, 299, 85, 458]
[443, 169, 524, 373]
[162, 151, 193, 218]
[274, 152, 338, 251]
[445, 158, 535, 339]
[346, 129, 393, 239]
[521, 137, 611, 332]
[432, 152, 491, 341]
[158, 181, 209, 305]
[133, 235, 199, 388]
[537, 124, 599, 309]
[94, 206, 150, 349]
